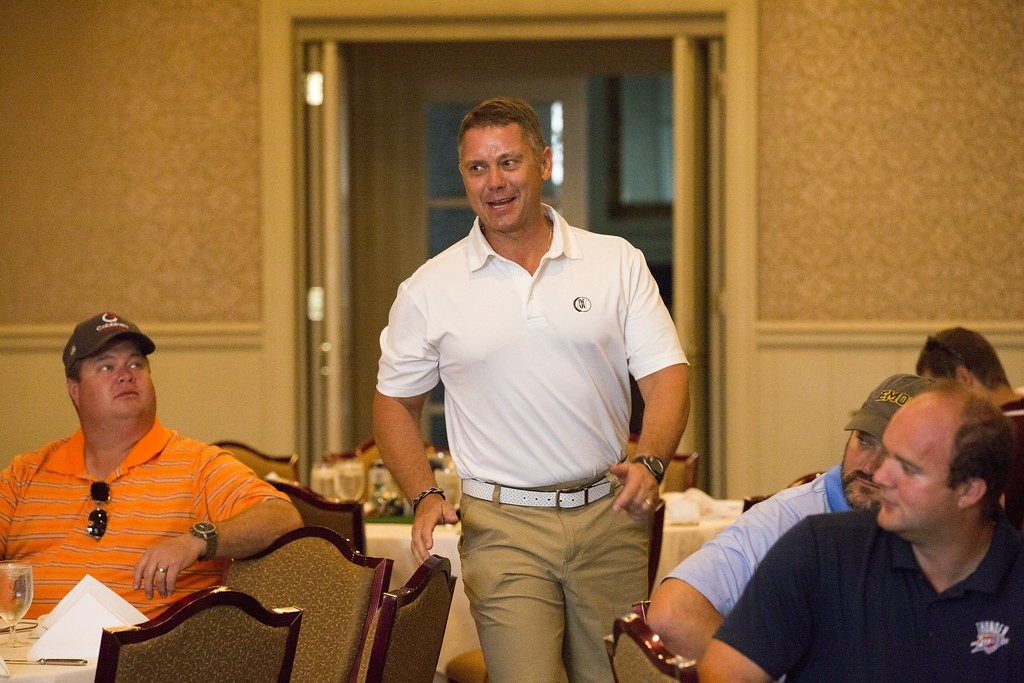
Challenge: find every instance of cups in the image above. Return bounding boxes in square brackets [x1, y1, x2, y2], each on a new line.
[311, 462, 337, 502]
[334, 461, 365, 502]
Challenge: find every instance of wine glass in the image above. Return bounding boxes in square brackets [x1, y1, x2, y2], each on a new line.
[0, 560, 34, 649]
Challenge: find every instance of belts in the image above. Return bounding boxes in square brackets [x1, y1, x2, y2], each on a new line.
[462, 456, 631, 511]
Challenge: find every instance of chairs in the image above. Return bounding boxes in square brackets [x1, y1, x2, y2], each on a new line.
[91, 435, 824, 683]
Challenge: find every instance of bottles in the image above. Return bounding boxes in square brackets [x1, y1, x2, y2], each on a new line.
[371, 458, 385, 506]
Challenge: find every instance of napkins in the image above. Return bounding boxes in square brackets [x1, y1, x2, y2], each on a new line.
[28, 573, 150, 638]
[26, 590, 132, 661]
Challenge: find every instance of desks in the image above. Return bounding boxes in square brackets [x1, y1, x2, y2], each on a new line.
[0, 613, 142, 683]
[352, 488, 751, 638]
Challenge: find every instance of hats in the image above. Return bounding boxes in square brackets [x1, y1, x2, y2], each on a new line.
[61, 312, 156, 372]
[843, 374, 938, 440]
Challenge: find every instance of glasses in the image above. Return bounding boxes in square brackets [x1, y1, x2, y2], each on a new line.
[924, 334, 975, 375]
[88, 481, 110, 540]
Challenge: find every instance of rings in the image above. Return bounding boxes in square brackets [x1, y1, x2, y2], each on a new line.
[646, 498, 655, 507]
[158, 567, 168, 573]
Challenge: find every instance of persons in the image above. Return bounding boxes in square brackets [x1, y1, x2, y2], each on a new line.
[647, 374, 936, 664]
[372, 96, 693, 683]
[0, 311, 303, 621]
[697, 381, 1024, 683]
[916, 326, 1024, 443]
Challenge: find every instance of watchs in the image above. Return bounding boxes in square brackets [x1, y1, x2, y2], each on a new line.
[630, 456, 665, 485]
[191, 521, 219, 565]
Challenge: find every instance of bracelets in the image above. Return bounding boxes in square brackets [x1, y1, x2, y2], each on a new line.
[411, 488, 447, 514]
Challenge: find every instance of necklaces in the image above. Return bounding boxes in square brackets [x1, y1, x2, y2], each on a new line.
[544, 220, 553, 253]
